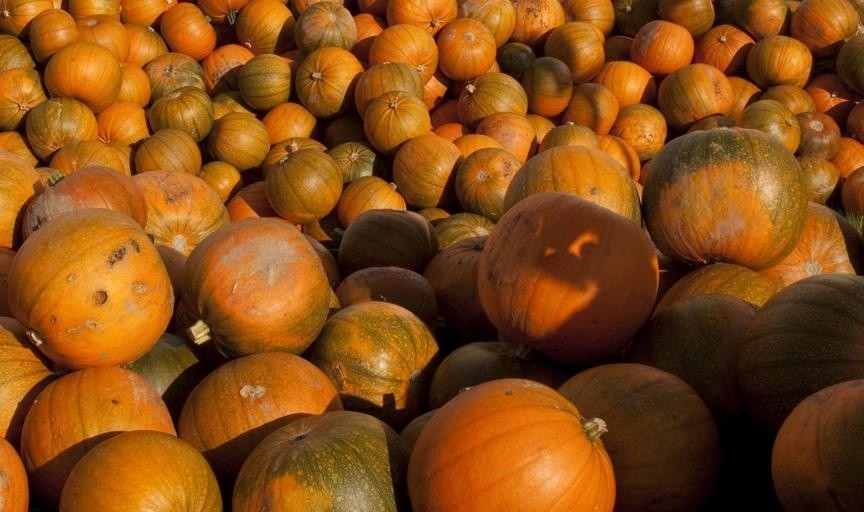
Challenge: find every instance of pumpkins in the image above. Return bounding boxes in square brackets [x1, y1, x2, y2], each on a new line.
[1, 1, 864, 512]
